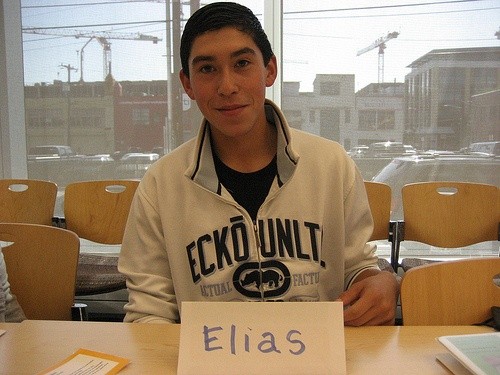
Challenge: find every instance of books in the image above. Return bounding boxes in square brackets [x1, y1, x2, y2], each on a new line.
[436, 332, 500, 375]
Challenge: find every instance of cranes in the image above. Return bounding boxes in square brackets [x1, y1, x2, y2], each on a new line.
[355, 31, 399, 86]
[22, 28, 163, 81]
[62, 65, 78, 84]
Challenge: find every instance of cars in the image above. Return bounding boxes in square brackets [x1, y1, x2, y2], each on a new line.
[25, 143, 162, 177]
[347, 140, 500, 162]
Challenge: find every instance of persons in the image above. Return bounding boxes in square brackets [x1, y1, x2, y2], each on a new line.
[117, 2, 400, 327]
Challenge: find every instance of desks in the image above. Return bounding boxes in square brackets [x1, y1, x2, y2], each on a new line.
[0, 320, 500, 375]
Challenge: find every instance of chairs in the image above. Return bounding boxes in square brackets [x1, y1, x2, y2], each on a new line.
[0, 179, 500, 326]
